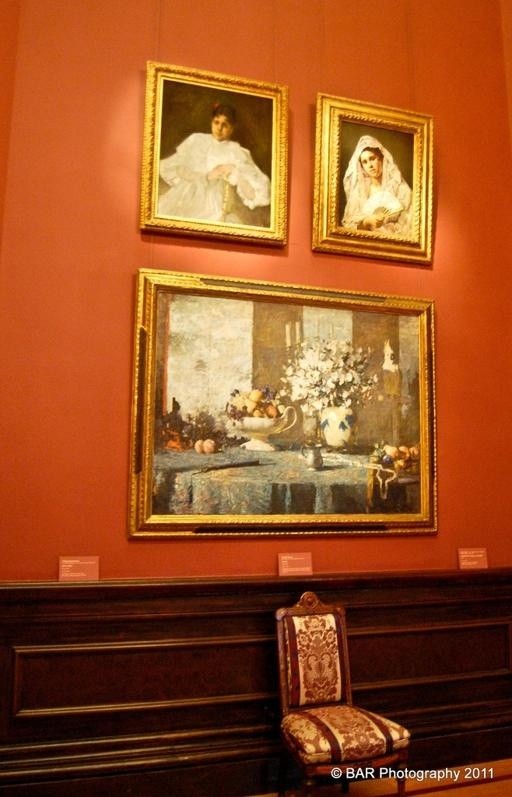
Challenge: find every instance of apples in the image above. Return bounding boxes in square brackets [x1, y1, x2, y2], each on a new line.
[195, 439, 205, 454]
[203, 439, 217, 454]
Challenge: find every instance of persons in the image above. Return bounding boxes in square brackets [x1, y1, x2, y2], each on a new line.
[341, 134, 412, 236]
[155, 103, 271, 226]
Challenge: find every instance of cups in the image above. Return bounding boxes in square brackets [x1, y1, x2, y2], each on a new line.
[301, 442, 324, 473]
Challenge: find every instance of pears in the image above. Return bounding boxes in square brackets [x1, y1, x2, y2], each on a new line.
[244, 389, 278, 418]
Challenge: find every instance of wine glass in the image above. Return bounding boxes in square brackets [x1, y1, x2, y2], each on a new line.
[231, 405, 298, 452]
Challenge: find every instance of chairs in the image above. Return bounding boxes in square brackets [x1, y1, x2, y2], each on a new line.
[272, 587, 413, 796]
